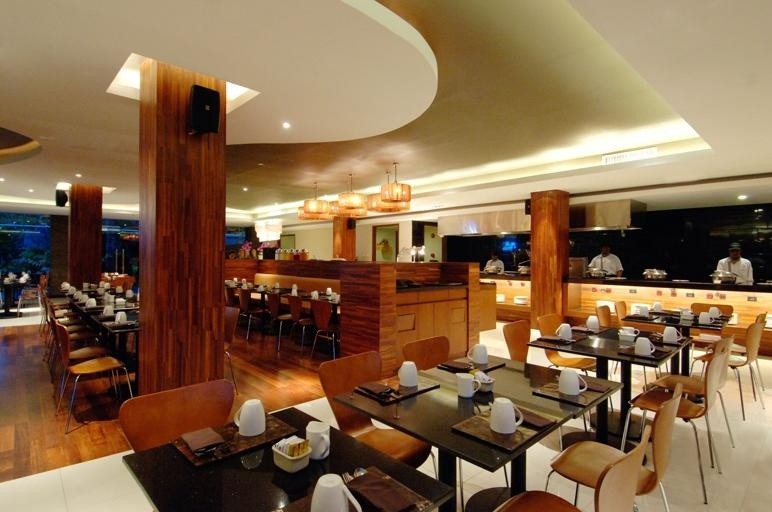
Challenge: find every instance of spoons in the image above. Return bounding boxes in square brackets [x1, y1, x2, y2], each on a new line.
[354, 468, 367, 477]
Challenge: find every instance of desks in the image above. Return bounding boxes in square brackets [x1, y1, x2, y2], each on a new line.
[121, 407, 455, 512]
[568, 280, 768, 301]
[483, 274, 532, 302]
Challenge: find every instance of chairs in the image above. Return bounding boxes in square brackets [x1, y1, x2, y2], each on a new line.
[18, 275, 133, 434]
[319, 302, 769, 511]
[121, 378, 235, 452]
[224, 281, 336, 360]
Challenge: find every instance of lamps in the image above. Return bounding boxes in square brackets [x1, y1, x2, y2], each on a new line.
[367, 194, 382, 211]
[380, 161, 411, 202]
[327, 200, 339, 215]
[297, 207, 332, 220]
[338, 172, 364, 210]
[377, 172, 410, 212]
[303, 180, 331, 214]
[336, 207, 367, 218]
[252, 215, 284, 242]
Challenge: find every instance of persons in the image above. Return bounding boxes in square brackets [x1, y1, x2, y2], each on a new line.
[483, 253, 504, 275]
[716, 243, 754, 286]
[588, 242, 624, 277]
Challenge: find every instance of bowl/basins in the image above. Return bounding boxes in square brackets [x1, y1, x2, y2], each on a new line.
[270, 442, 312, 473]
[709, 274, 737, 283]
[585, 271, 607, 278]
[474, 377, 495, 392]
[641, 272, 668, 280]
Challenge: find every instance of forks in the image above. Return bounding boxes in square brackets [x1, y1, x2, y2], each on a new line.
[341, 472, 353, 482]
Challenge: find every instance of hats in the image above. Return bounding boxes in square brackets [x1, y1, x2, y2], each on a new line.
[728, 243, 741, 250]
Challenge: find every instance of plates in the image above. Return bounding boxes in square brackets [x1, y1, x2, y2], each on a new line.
[514, 296, 528, 305]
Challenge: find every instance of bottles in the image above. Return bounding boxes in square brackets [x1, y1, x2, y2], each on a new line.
[274, 247, 309, 261]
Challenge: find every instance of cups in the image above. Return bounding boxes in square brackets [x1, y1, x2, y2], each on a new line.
[226, 277, 280, 292]
[234, 398, 266, 437]
[60, 280, 134, 308]
[680, 306, 724, 324]
[466, 344, 489, 365]
[662, 327, 683, 343]
[305, 420, 331, 461]
[555, 323, 572, 338]
[116, 312, 127, 325]
[559, 368, 587, 396]
[455, 372, 481, 398]
[397, 360, 419, 387]
[309, 474, 363, 512]
[617, 326, 639, 342]
[638, 302, 662, 317]
[292, 284, 298, 296]
[103, 305, 114, 315]
[240, 449, 265, 469]
[311, 287, 340, 302]
[586, 316, 598, 328]
[635, 337, 655, 355]
[489, 398, 524, 433]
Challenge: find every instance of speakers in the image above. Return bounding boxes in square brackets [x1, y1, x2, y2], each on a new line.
[524, 199, 531, 214]
[55, 190, 69, 207]
[347, 218, 356, 230]
[187, 84, 220, 133]
